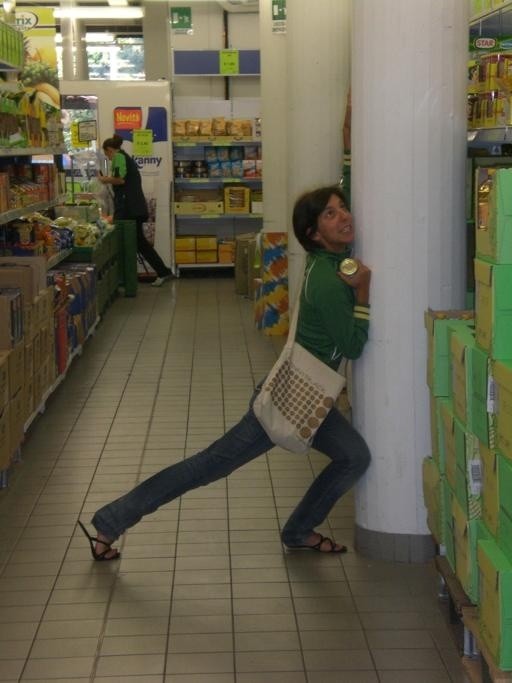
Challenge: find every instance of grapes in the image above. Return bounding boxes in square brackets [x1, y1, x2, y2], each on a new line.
[19, 61, 59, 88]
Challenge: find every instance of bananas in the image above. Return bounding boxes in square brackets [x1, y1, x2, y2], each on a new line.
[27, 82, 60, 109]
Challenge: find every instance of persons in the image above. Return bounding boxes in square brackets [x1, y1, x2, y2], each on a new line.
[96, 135, 176, 287]
[76, 185, 372, 560]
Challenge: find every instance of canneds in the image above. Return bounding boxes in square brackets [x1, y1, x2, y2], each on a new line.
[339, 258, 359, 277]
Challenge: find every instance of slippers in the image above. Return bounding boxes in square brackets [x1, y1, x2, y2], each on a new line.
[75, 518, 121, 562]
[284, 534, 349, 554]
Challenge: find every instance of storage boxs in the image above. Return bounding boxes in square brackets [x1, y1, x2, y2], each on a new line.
[418, 4, 512, 682]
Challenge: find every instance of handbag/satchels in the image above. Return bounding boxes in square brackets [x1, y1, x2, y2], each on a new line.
[252, 342, 346, 456]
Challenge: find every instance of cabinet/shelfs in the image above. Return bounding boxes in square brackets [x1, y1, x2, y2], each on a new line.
[171, 134, 260, 280]
[3, 2, 119, 486]
